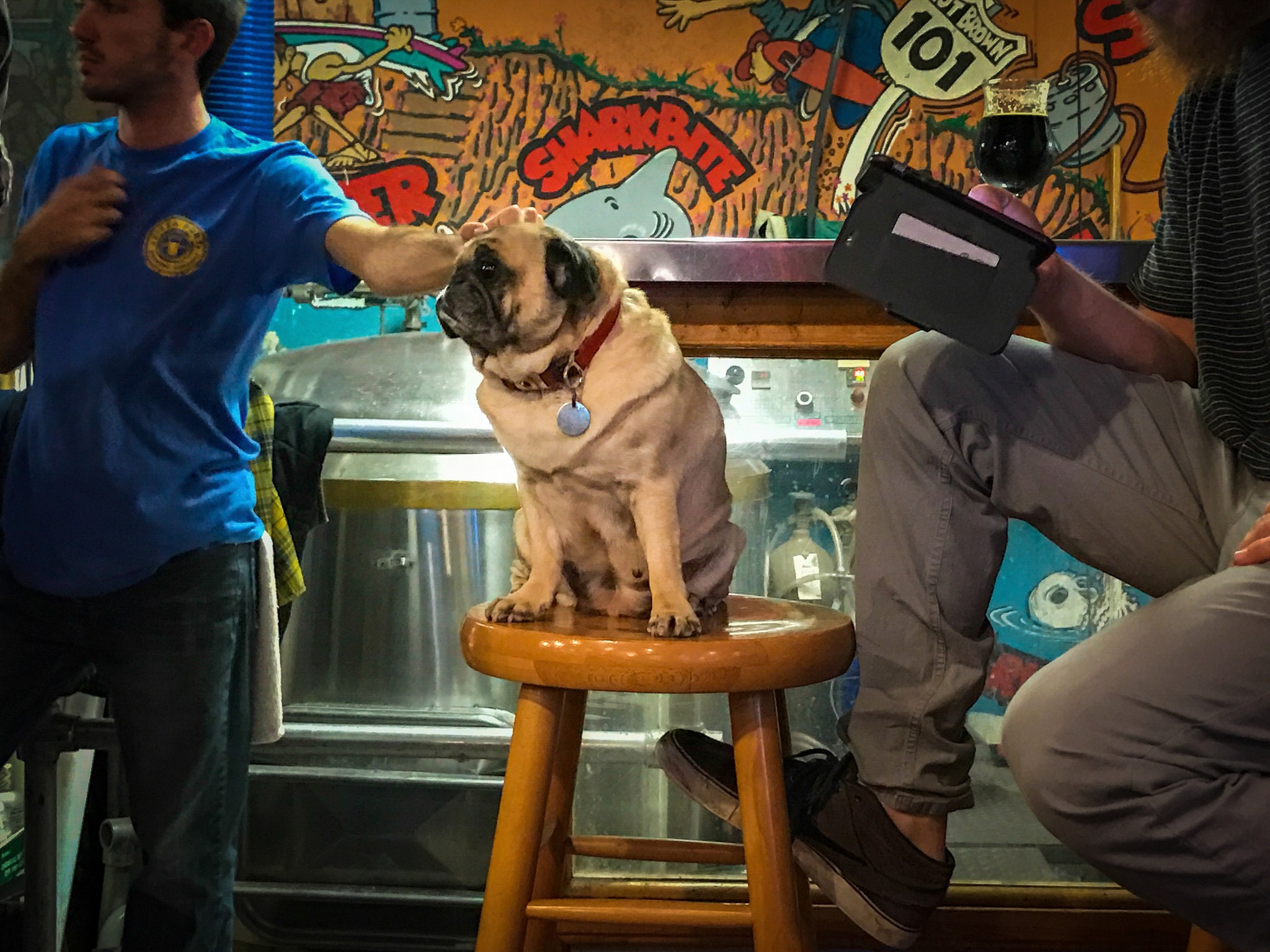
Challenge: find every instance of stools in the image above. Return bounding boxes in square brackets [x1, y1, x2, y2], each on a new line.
[458, 591, 859, 952]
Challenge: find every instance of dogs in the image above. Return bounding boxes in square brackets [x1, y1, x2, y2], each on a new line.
[436, 222, 748, 639]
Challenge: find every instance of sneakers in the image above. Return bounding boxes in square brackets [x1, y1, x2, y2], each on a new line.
[654, 729, 956, 950]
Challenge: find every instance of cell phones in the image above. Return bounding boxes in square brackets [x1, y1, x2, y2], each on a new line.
[821, 153, 1058, 356]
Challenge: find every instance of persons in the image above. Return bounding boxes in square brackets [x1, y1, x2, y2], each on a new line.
[655, 3, 1267, 952]
[2, 1, 548, 952]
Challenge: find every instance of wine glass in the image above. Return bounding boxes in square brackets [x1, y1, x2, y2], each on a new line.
[973, 77, 1060, 199]
